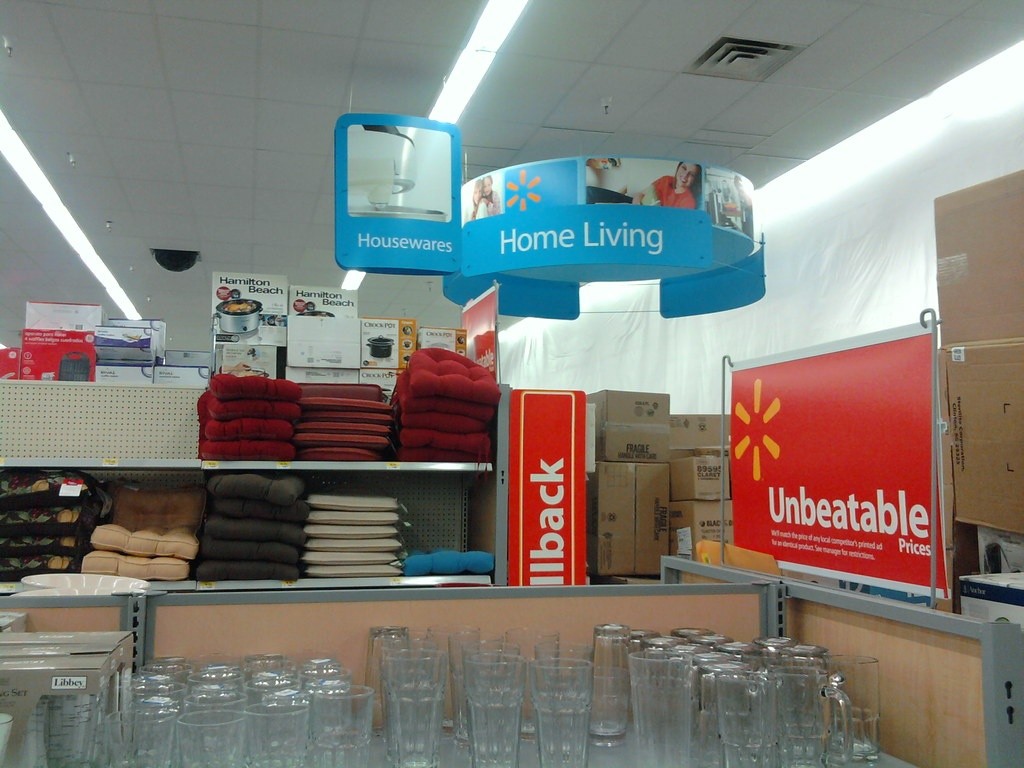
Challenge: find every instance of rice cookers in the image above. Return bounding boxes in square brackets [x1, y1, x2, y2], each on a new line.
[212, 299, 263, 333]
[366, 335, 395, 358]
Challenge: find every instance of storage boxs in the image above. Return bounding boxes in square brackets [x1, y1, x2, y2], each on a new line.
[672, 449, 694, 460]
[0, 610, 133, 768]
[671, 500, 733, 555]
[670, 457, 731, 501]
[977, 526, 1024, 574]
[934, 169, 1024, 348]
[958, 572, 1024, 629]
[668, 413, 730, 449]
[695, 448, 721, 458]
[636, 463, 671, 576]
[587, 463, 636, 577]
[0, 272, 469, 395]
[586, 390, 672, 463]
[945, 342, 1024, 536]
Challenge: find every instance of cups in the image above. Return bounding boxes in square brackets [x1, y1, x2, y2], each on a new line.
[0, 713, 14, 766]
[105, 624, 880, 768]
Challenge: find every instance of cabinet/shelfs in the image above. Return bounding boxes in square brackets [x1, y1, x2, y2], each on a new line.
[0, 378, 512, 594]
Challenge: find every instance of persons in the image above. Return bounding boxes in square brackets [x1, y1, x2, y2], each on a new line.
[714, 175, 754, 241]
[631, 159, 703, 210]
[470, 179, 489, 221]
[480, 175, 502, 217]
